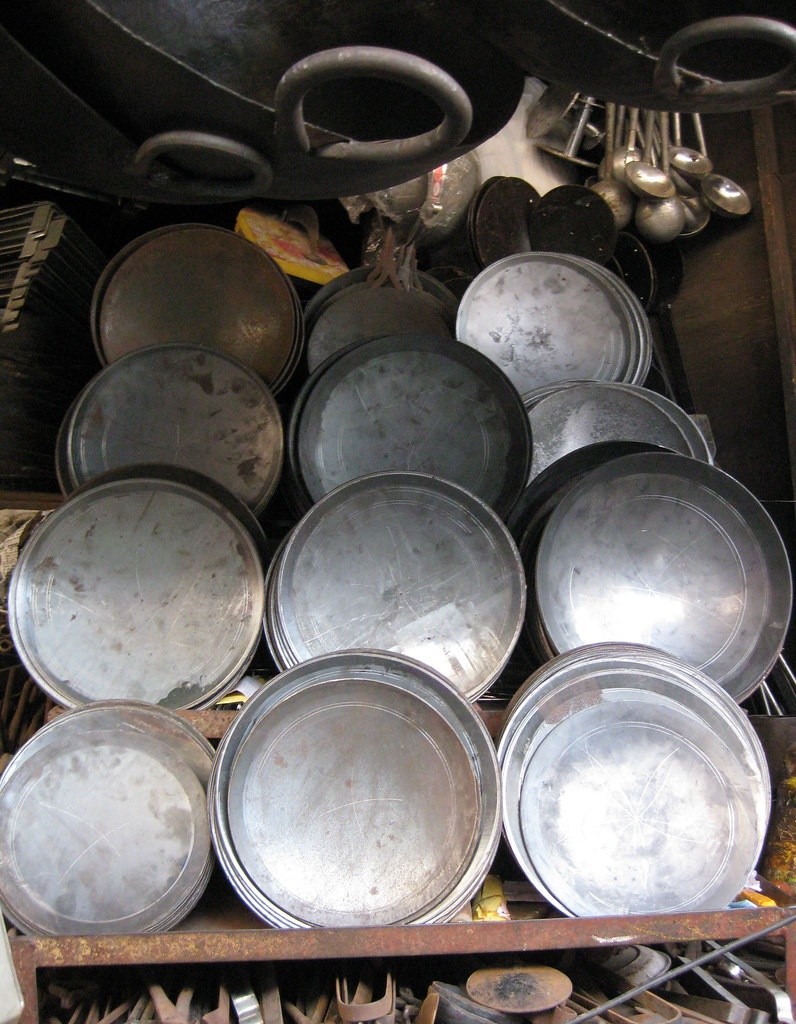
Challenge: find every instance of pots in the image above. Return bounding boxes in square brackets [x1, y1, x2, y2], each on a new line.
[0, 23, 275, 208]
[408, 0, 796, 112]
[0, 0, 527, 200]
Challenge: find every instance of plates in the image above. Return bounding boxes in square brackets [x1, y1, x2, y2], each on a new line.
[1, 223, 794, 934]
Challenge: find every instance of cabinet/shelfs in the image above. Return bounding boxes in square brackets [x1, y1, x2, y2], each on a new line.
[0, 490, 796, 1024]
